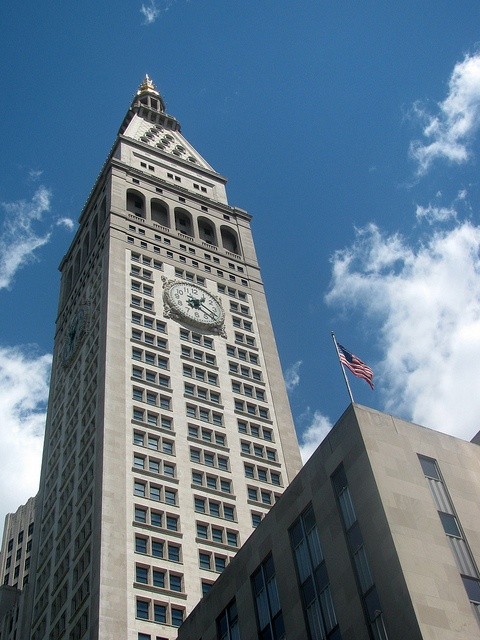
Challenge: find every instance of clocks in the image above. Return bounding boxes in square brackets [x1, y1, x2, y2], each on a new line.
[169, 282, 223, 325]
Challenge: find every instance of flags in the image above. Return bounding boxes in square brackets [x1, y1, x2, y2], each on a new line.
[337, 342, 375, 390]
